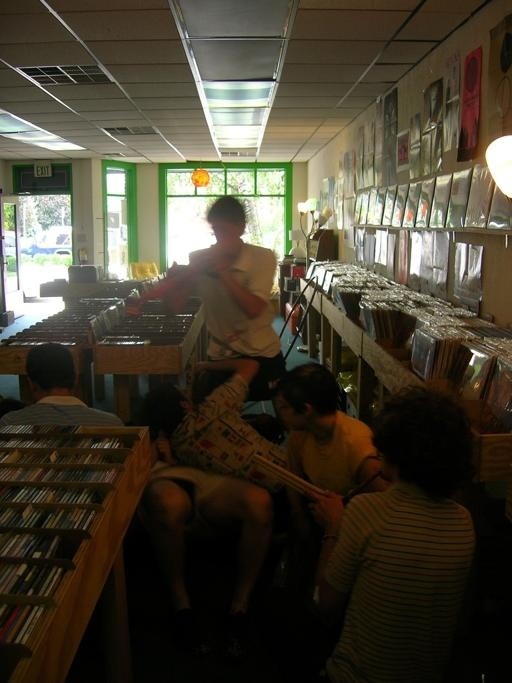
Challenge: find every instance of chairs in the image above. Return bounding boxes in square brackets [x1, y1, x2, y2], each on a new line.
[131, 261, 158, 282]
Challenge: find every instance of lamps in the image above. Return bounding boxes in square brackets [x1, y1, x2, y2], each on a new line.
[191, 159, 210, 187]
[289, 229, 306, 257]
